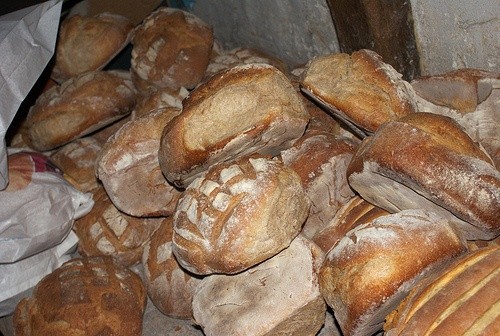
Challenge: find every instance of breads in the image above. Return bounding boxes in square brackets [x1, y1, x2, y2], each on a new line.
[13, 0, 500, 336]
